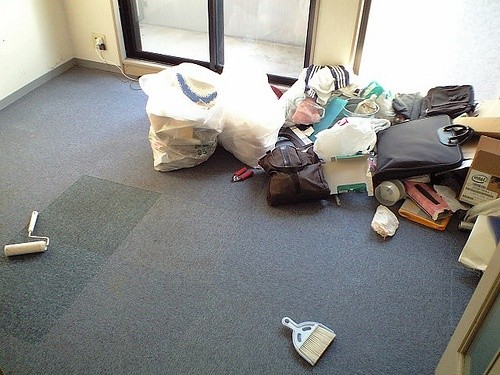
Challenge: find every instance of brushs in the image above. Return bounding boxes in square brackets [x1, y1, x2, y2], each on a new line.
[3, 211, 50, 256]
[281, 316, 337, 367]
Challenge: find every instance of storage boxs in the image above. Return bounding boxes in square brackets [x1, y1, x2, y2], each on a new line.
[458, 133, 500, 210]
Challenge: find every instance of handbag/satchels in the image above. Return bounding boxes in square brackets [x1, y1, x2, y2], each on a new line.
[215, 58, 284, 169]
[258, 144, 331, 206]
[374, 114, 473, 182]
[312, 117, 391, 160]
[418, 84, 476, 118]
[138, 63, 226, 173]
[279, 62, 360, 128]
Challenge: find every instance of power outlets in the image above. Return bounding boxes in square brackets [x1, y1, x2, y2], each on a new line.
[91, 32, 105, 51]
